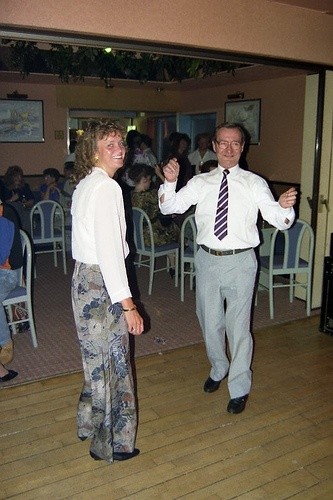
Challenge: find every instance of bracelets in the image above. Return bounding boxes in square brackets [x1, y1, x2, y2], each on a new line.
[122, 305, 137, 312]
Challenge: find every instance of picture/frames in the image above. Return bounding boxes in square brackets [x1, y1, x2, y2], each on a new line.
[0, 98, 45, 144]
[223, 98, 262, 145]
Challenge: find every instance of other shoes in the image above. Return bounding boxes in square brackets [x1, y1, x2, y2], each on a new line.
[0, 370, 18, 382]
[89, 451, 102, 460]
[0, 339, 14, 365]
[113, 449, 139, 460]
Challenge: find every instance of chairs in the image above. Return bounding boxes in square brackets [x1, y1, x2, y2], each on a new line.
[2, 185, 315, 349]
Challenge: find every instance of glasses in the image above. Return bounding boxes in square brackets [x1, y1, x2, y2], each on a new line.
[214, 139, 242, 149]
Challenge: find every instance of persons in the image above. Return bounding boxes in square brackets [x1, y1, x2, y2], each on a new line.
[110, 130, 218, 280]
[70, 117, 145, 464]
[158, 121, 299, 414]
[0, 161, 78, 382]
[69, 129, 77, 153]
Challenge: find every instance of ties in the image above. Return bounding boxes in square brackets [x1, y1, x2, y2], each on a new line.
[213, 169, 230, 241]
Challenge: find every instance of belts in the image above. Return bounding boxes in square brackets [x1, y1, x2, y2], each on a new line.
[201, 245, 250, 256]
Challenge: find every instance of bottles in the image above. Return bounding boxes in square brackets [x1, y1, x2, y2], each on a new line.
[22, 195, 27, 210]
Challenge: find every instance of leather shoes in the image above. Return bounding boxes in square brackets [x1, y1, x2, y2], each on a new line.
[204, 373, 228, 392]
[17, 321, 29, 333]
[227, 395, 247, 414]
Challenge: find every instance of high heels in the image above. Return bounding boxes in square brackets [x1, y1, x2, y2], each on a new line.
[170, 268, 185, 280]
